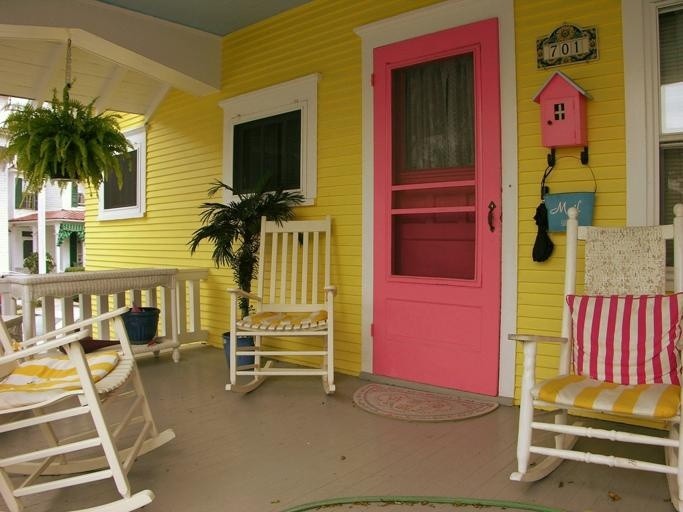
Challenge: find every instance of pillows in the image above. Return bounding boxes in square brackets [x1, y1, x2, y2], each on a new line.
[563, 289, 683, 387]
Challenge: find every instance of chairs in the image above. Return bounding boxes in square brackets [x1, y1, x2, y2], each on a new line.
[509, 205, 683, 510]
[0, 306, 175, 511]
[225, 216, 335, 394]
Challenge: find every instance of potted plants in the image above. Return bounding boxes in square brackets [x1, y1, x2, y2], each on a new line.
[0, 80, 132, 209]
[188, 173, 304, 370]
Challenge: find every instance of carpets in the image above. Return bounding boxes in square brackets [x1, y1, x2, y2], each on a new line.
[351, 381, 501, 421]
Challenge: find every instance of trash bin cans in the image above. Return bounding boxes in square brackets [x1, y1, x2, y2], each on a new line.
[222, 332, 254, 371]
